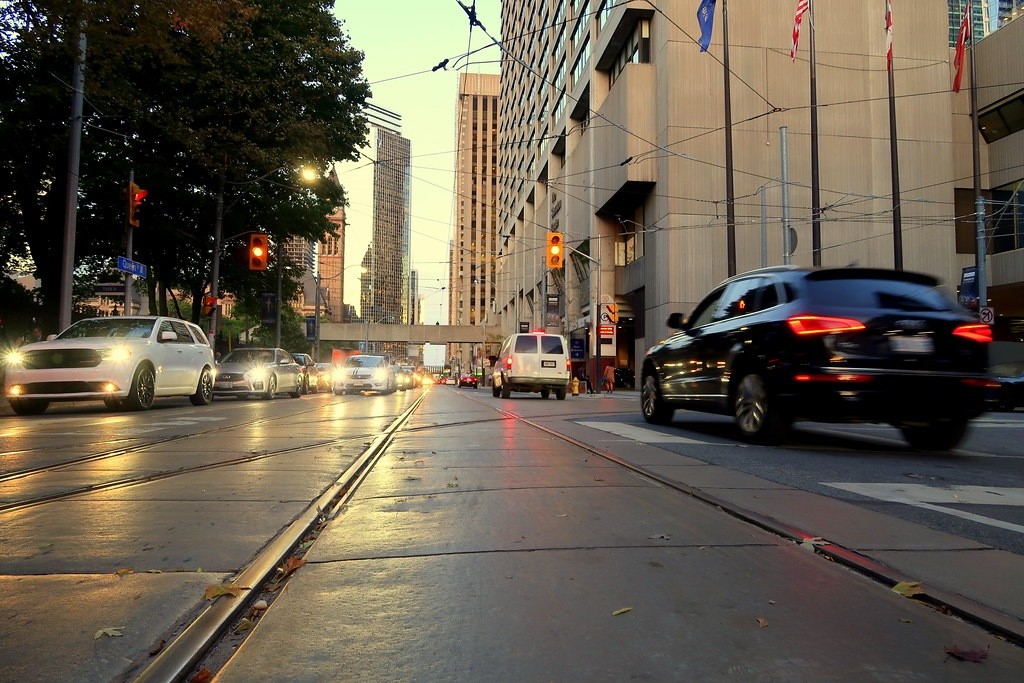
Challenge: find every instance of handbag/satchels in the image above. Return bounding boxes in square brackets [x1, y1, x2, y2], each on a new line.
[602, 376, 607, 385]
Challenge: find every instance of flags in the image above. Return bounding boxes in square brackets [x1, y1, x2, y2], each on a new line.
[790, 0, 808, 63]
[884, 0, 893, 72]
[952, 0, 972, 93]
[697, 0, 716, 52]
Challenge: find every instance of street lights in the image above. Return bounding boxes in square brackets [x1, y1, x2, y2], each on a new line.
[207, 164, 316, 349]
[366, 316, 395, 353]
[474, 278, 521, 332]
[315, 264, 367, 361]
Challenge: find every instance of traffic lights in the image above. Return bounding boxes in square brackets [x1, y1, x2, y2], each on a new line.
[203, 295, 217, 315]
[546, 232, 563, 269]
[249, 234, 267, 270]
[606, 304, 618, 323]
[129, 181, 148, 228]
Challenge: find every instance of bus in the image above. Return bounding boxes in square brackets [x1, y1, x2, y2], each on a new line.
[433, 374, 443, 381]
[402, 366, 416, 372]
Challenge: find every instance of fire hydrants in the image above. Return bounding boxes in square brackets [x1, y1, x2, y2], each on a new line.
[571, 377, 580, 396]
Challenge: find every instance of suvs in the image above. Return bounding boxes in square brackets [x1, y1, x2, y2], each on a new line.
[640, 265, 997, 453]
[4, 314, 218, 411]
[489, 332, 570, 400]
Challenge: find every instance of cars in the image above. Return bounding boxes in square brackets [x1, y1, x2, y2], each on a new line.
[438, 377, 456, 385]
[334, 355, 415, 395]
[315, 363, 334, 392]
[289, 352, 319, 393]
[216, 347, 304, 401]
[457, 373, 478, 389]
[985, 361, 1024, 412]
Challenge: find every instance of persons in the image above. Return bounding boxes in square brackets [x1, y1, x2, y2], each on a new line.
[215, 351, 222, 364]
[603, 362, 615, 393]
[579, 371, 595, 394]
[30, 326, 46, 343]
